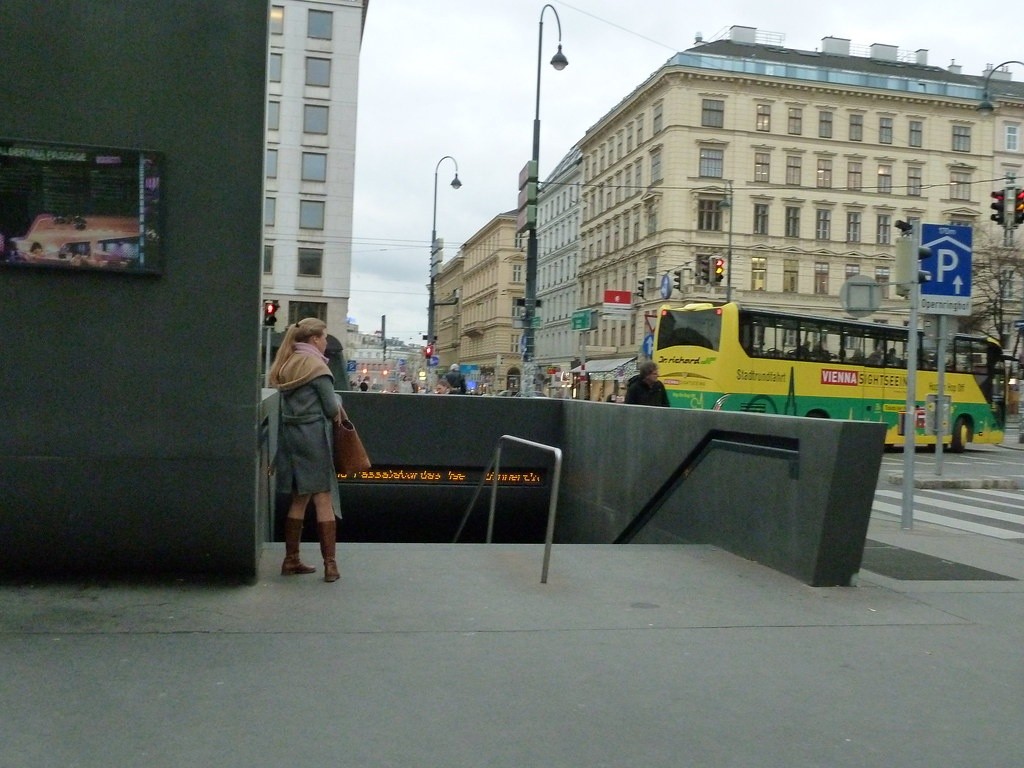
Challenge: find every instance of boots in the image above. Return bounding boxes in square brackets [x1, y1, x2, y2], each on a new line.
[316, 520, 342, 582]
[281, 517, 317, 575]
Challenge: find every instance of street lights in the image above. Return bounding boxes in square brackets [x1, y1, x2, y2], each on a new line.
[425, 156, 463, 394]
[520, 3, 569, 397]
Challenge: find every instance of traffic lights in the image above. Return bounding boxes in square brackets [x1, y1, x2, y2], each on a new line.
[703, 256, 724, 285]
[1015, 188, 1024, 225]
[425, 346, 430, 360]
[637, 278, 648, 300]
[673, 268, 685, 294]
[264, 301, 278, 328]
[917, 246, 933, 282]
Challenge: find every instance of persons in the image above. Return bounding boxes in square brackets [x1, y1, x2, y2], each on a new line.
[349, 364, 617, 402]
[267, 319, 343, 582]
[798, 342, 964, 370]
[624, 361, 671, 407]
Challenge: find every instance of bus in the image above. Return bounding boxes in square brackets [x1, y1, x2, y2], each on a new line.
[652, 305, 1006, 454]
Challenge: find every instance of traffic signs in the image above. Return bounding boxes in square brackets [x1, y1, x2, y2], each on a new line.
[990, 189, 1004, 226]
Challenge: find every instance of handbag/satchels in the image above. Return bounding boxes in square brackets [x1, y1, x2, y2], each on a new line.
[332, 400, 371, 475]
